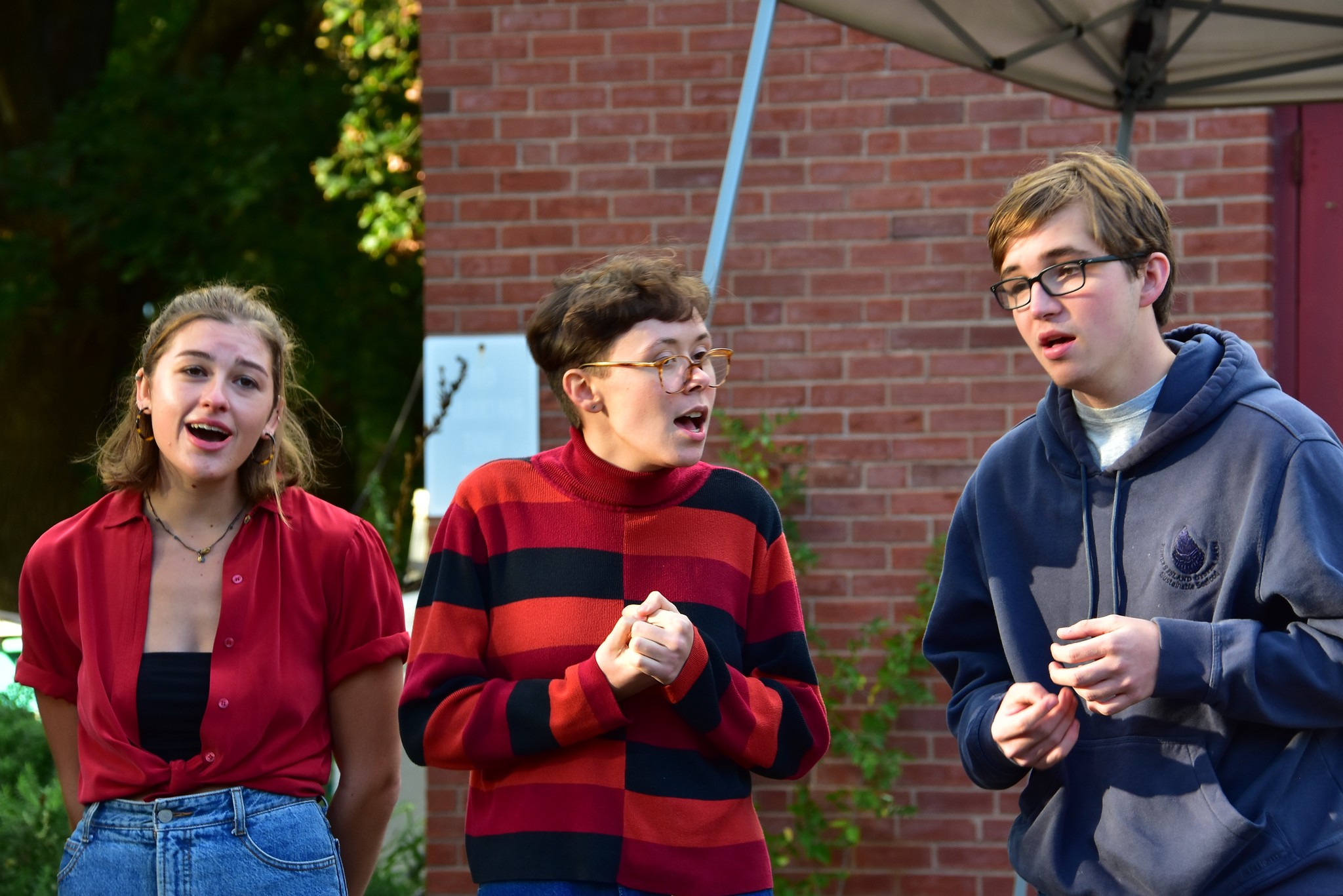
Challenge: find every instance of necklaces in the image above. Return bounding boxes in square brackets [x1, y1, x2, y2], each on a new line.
[142, 486, 247, 564]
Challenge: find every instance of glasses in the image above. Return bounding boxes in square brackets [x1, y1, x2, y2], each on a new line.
[580, 347, 733, 396]
[989, 251, 1150, 311]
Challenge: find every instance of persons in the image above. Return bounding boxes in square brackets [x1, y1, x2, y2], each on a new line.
[925, 149, 1342, 896]
[399, 249, 830, 896]
[18, 280, 408, 896]
